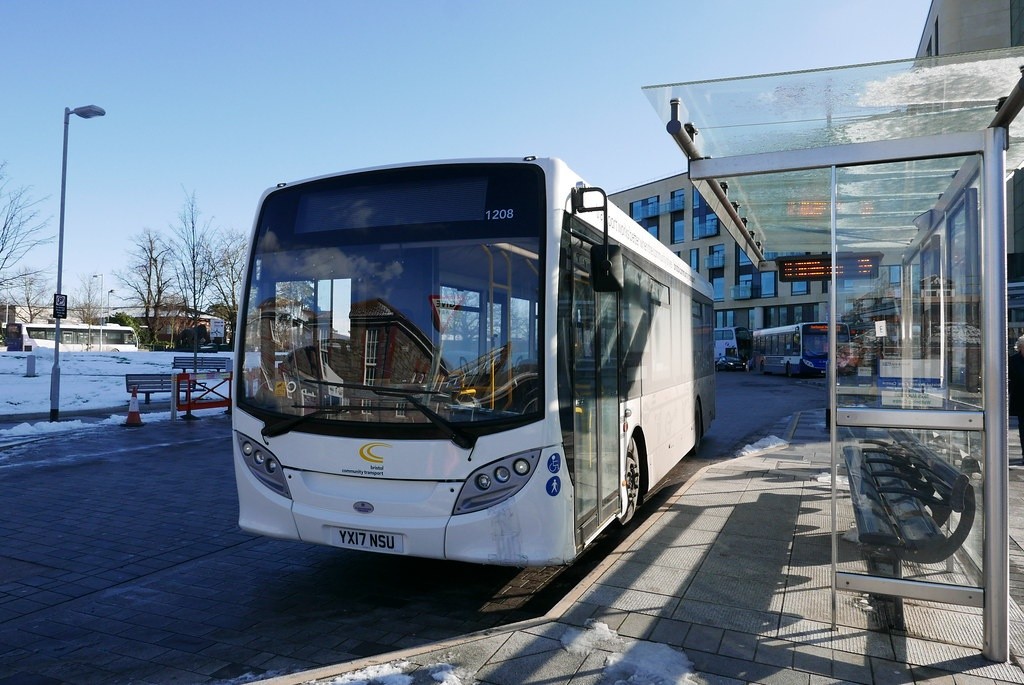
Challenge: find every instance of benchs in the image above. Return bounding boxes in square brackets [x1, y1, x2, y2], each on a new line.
[843, 427, 975, 632]
[126, 373, 207, 403]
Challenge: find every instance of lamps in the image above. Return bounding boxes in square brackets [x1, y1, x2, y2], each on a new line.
[912, 209, 933, 230]
[173, 356, 230, 374]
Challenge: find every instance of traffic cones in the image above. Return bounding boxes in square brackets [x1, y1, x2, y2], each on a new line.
[120, 387, 147, 427]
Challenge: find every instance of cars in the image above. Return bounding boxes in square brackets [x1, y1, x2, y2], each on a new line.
[715, 356, 751, 372]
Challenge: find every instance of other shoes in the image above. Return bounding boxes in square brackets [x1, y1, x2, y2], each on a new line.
[1021, 457, 1024, 465]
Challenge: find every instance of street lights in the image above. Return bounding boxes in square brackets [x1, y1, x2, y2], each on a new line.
[93, 273, 102, 353]
[50, 104, 105, 422]
[108, 289, 115, 323]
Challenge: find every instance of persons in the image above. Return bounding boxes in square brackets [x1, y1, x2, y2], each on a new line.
[1008, 332, 1024, 461]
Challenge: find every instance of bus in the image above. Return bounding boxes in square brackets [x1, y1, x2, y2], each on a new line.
[5, 321, 139, 352]
[752, 324, 852, 377]
[714, 327, 752, 361]
[232, 152, 716, 567]
